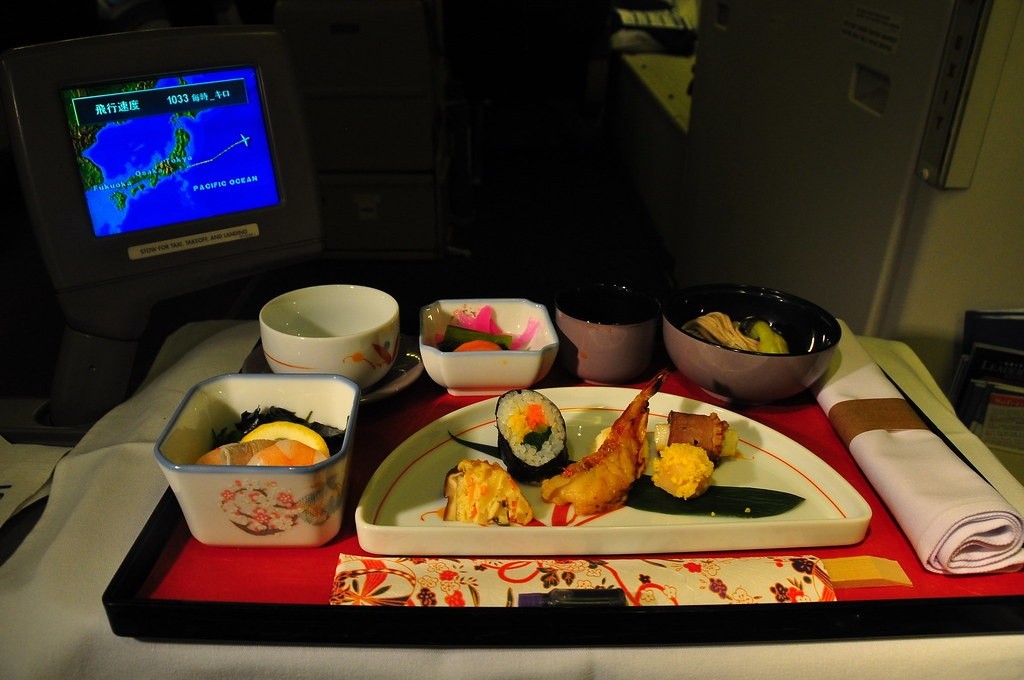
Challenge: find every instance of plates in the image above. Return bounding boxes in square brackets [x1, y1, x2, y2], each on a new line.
[355, 387, 873, 556]
[242, 346, 425, 402]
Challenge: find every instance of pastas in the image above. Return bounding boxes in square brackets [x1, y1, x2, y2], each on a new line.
[681, 311, 759, 352]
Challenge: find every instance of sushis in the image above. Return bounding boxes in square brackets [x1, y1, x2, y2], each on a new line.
[495, 388, 569, 483]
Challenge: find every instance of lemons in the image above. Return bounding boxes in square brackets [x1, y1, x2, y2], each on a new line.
[241, 421, 330, 460]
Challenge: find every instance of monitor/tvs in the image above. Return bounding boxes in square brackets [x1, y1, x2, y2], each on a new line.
[5, 27, 323, 288]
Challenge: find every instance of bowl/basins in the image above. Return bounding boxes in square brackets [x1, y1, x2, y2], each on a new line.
[153, 374, 362, 546]
[418, 299, 559, 396]
[259, 283, 400, 388]
[661, 283, 843, 403]
[554, 283, 659, 386]
